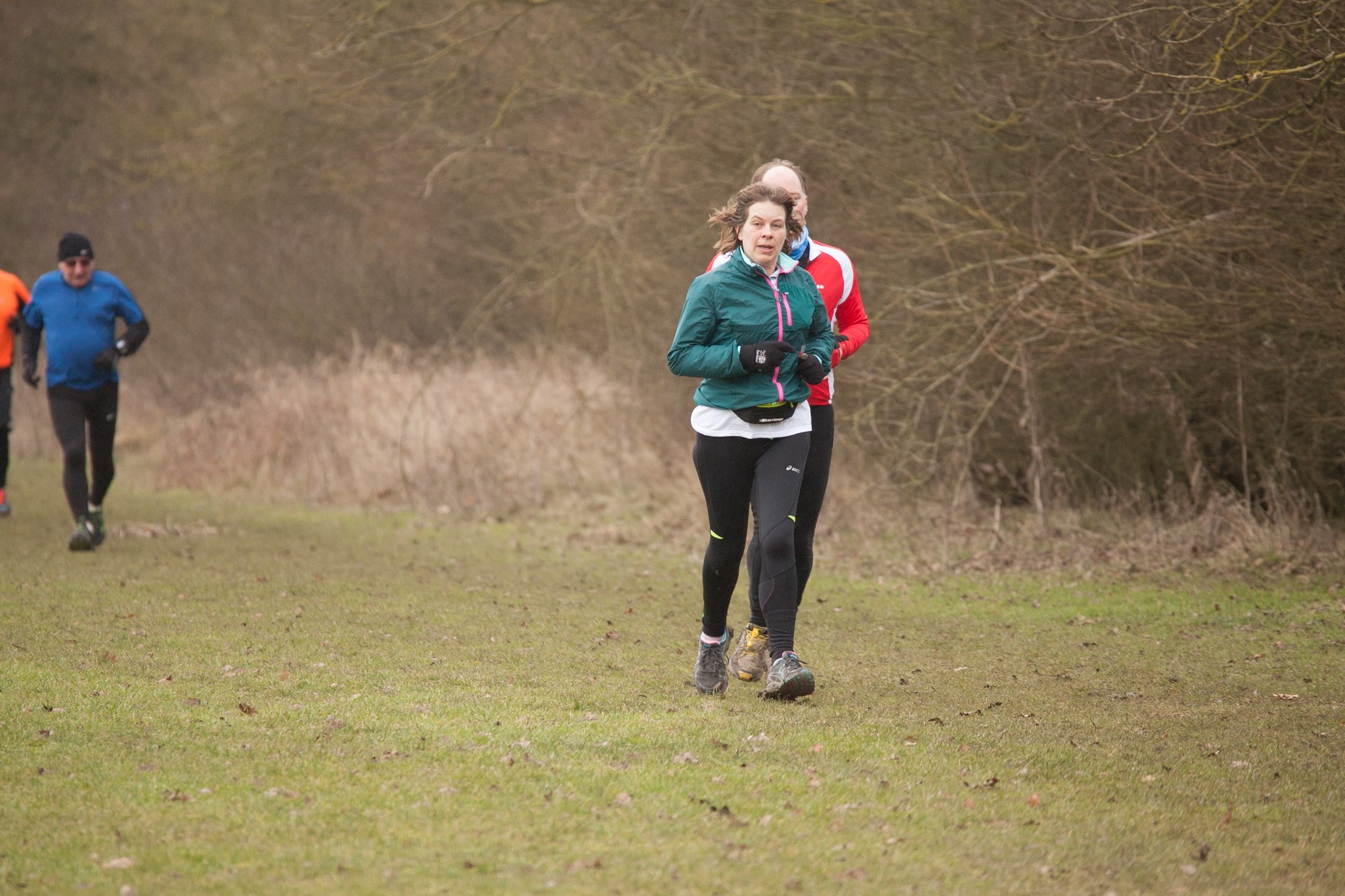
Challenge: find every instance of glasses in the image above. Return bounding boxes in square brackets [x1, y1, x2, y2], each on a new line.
[60, 257, 95, 268]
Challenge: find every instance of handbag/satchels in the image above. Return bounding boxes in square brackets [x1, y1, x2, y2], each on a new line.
[727, 398, 800, 425]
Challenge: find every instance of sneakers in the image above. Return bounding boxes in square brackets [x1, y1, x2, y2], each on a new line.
[727, 618, 772, 681]
[0, 489, 11, 516]
[68, 523, 94, 550]
[759, 649, 816, 702]
[85, 496, 105, 546]
[693, 632, 730, 698]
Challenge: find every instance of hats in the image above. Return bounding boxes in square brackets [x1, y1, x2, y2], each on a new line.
[56, 232, 96, 261]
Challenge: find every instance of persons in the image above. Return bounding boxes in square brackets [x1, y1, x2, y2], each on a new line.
[18, 231, 152, 554]
[699, 153, 868, 685]
[663, 182, 838, 701]
[0, 269, 35, 517]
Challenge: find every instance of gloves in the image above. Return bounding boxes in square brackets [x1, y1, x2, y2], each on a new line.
[6, 314, 25, 335]
[798, 351, 825, 385]
[22, 368, 40, 388]
[738, 339, 796, 373]
[92, 345, 120, 375]
